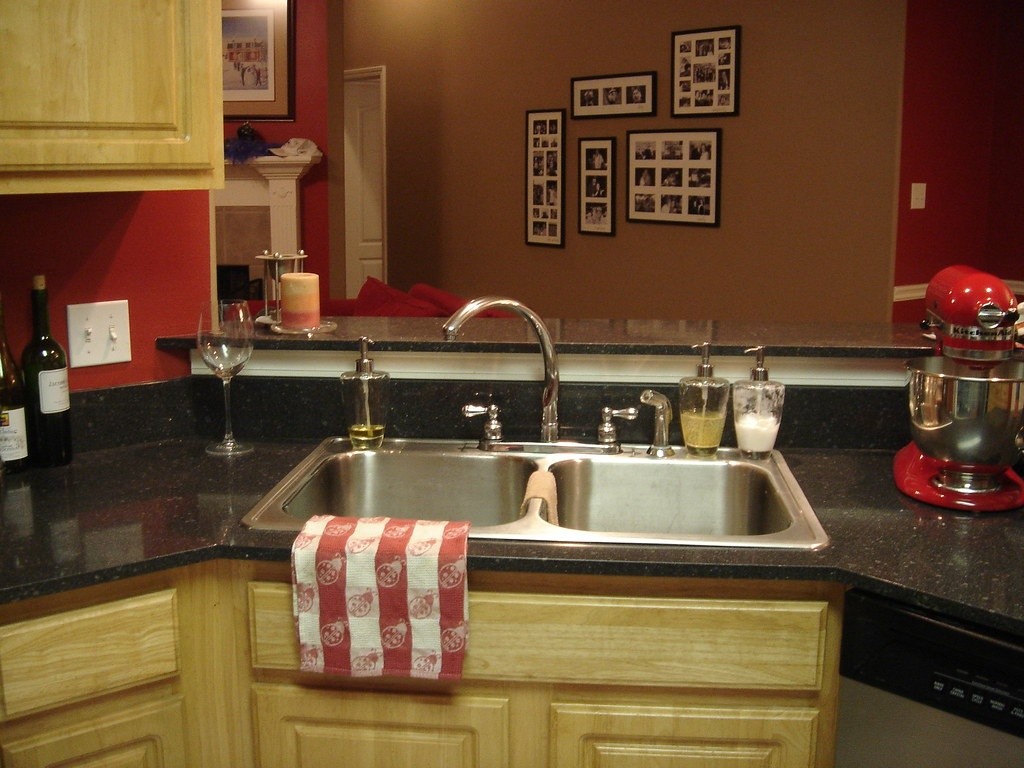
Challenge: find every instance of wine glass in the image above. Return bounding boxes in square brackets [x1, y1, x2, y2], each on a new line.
[197, 299, 253, 456]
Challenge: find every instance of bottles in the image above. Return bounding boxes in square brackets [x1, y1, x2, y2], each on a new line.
[0, 324, 31, 472]
[20, 273, 73, 467]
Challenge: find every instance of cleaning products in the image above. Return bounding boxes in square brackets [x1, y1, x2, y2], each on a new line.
[678, 342, 785, 460]
[338, 335, 392, 448]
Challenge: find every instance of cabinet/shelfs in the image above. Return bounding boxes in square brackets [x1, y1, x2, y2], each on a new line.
[0, 557, 846, 768]
[0, 0, 225, 195]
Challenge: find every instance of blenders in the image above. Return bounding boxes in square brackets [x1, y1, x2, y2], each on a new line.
[892, 265, 1024, 508]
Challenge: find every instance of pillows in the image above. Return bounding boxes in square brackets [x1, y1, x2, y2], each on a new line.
[349, 277, 439, 317]
[412, 284, 519, 317]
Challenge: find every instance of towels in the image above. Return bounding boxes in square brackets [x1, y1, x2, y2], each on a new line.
[265, 136, 325, 158]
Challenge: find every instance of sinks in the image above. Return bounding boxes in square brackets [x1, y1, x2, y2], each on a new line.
[534, 456, 797, 538]
[283, 446, 537, 531]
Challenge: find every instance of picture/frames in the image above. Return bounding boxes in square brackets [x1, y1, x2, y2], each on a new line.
[525, 109, 564, 249]
[671, 25, 741, 118]
[625, 128, 722, 227]
[576, 136, 616, 237]
[570, 72, 657, 118]
[222, 0, 297, 123]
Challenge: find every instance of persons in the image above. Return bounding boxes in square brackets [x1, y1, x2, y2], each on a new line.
[635, 194, 705, 215]
[689, 169, 700, 186]
[632, 87, 641, 103]
[679, 42, 691, 106]
[588, 177, 606, 197]
[591, 149, 605, 170]
[639, 169, 651, 186]
[642, 148, 652, 159]
[663, 171, 678, 187]
[698, 143, 710, 160]
[719, 38, 730, 107]
[585, 206, 606, 226]
[232, 60, 263, 88]
[608, 87, 617, 103]
[533, 121, 557, 236]
[583, 89, 594, 106]
[695, 40, 713, 106]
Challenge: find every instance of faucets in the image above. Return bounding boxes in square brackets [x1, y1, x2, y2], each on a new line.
[442, 293, 561, 431]
